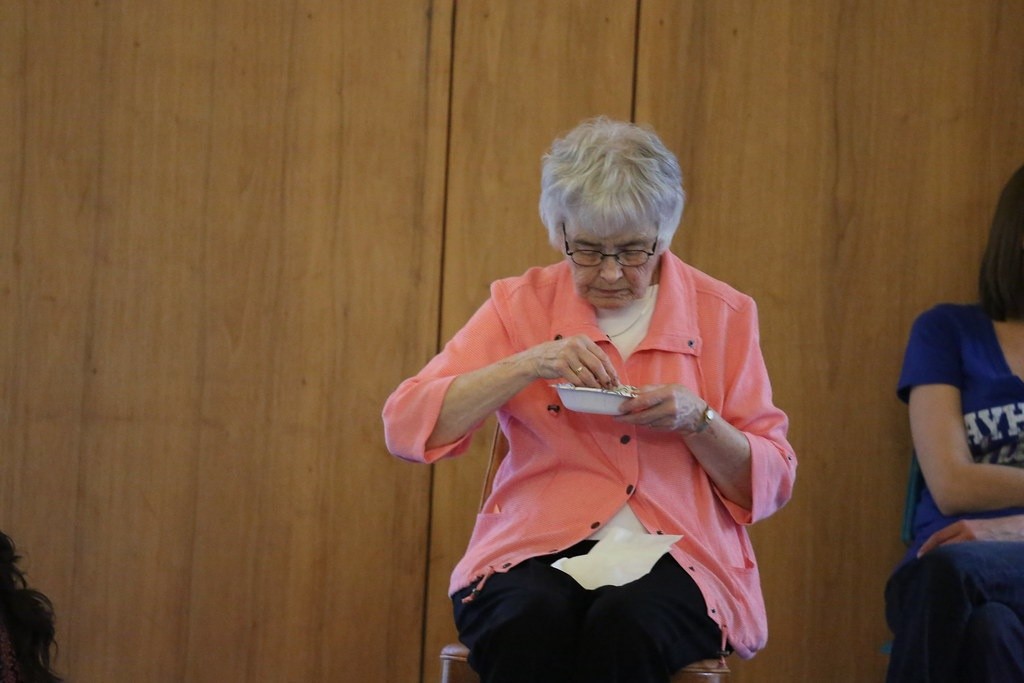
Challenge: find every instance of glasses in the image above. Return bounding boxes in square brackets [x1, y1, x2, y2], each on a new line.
[562, 222, 659, 266]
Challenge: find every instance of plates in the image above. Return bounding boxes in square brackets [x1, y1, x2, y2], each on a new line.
[550, 381, 635, 415]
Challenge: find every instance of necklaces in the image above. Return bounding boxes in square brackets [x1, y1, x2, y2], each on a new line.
[610, 275, 655, 340]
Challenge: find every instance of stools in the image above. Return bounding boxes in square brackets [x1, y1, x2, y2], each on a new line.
[441, 642, 730, 683]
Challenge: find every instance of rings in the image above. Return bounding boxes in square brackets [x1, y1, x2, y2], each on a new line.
[574, 364, 584, 374]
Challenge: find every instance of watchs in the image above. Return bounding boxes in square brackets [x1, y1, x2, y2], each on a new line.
[688, 403, 717, 438]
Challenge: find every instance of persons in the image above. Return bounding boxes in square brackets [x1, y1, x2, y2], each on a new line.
[381, 117, 798, 683]
[883, 167, 1024, 683]
[0, 532, 59, 683]
